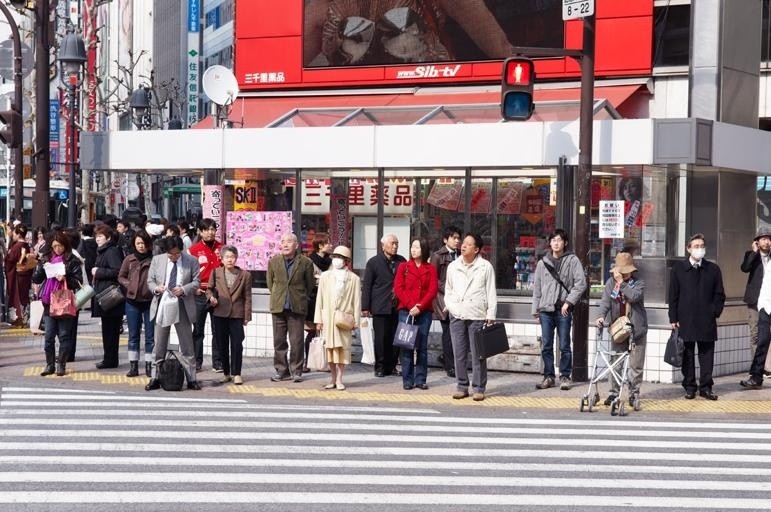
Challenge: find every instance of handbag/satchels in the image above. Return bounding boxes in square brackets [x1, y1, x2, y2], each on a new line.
[359, 313, 375, 365]
[158, 351, 185, 392]
[393, 314, 420, 349]
[474, 320, 510, 360]
[335, 312, 356, 331]
[306, 329, 328, 372]
[49, 285, 217, 327]
[607, 316, 634, 344]
[664, 326, 685, 368]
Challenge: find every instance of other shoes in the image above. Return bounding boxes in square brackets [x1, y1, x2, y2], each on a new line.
[56, 363, 67, 376]
[628, 395, 638, 406]
[145, 378, 161, 391]
[699, 391, 718, 401]
[604, 395, 617, 405]
[220, 375, 244, 385]
[740, 378, 760, 389]
[41, 362, 56, 377]
[324, 384, 346, 390]
[196, 365, 202, 372]
[535, 375, 556, 389]
[685, 392, 696, 399]
[404, 383, 428, 389]
[446, 371, 455, 378]
[212, 367, 224, 373]
[375, 370, 398, 377]
[452, 390, 469, 399]
[559, 375, 572, 389]
[187, 382, 202, 390]
[95, 361, 119, 369]
[473, 393, 484, 401]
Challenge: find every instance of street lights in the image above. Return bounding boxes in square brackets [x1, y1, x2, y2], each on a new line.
[58, 27, 87, 232]
[130, 79, 151, 208]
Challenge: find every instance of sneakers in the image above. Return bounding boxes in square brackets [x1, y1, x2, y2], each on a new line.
[271, 374, 292, 382]
[293, 372, 304, 382]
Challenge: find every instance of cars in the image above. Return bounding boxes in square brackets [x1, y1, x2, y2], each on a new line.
[481, 229, 491, 244]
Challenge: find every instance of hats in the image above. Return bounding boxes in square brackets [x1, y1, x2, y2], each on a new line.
[332, 246, 351, 259]
[609, 253, 638, 274]
[753, 226, 771, 239]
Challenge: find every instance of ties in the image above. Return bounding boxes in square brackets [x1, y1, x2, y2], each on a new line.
[168, 261, 176, 291]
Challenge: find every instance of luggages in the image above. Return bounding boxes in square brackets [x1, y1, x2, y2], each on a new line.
[28, 299, 46, 335]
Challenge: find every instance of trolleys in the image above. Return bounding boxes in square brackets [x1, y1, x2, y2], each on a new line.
[579, 318, 646, 418]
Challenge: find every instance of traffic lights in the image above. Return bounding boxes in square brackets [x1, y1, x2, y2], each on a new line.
[502, 56, 534, 120]
[0, 111, 13, 149]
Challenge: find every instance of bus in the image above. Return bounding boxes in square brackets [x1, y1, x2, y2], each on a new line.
[0, 176, 106, 239]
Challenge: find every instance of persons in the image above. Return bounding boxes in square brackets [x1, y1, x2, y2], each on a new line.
[312, 245, 363, 391]
[393, 236, 438, 390]
[264, 230, 313, 382]
[0, 211, 253, 391]
[618, 176, 642, 201]
[740, 261, 771, 388]
[303, 0, 516, 67]
[442, 232, 499, 401]
[668, 233, 726, 401]
[596, 251, 648, 404]
[361, 233, 407, 377]
[530, 228, 587, 391]
[740, 224, 771, 378]
[299, 231, 334, 372]
[430, 226, 464, 378]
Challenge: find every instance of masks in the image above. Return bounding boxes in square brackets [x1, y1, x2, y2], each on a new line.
[690, 248, 705, 258]
[332, 258, 345, 269]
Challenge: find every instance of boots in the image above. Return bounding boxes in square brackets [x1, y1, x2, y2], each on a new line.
[126, 362, 139, 377]
[145, 361, 152, 378]
